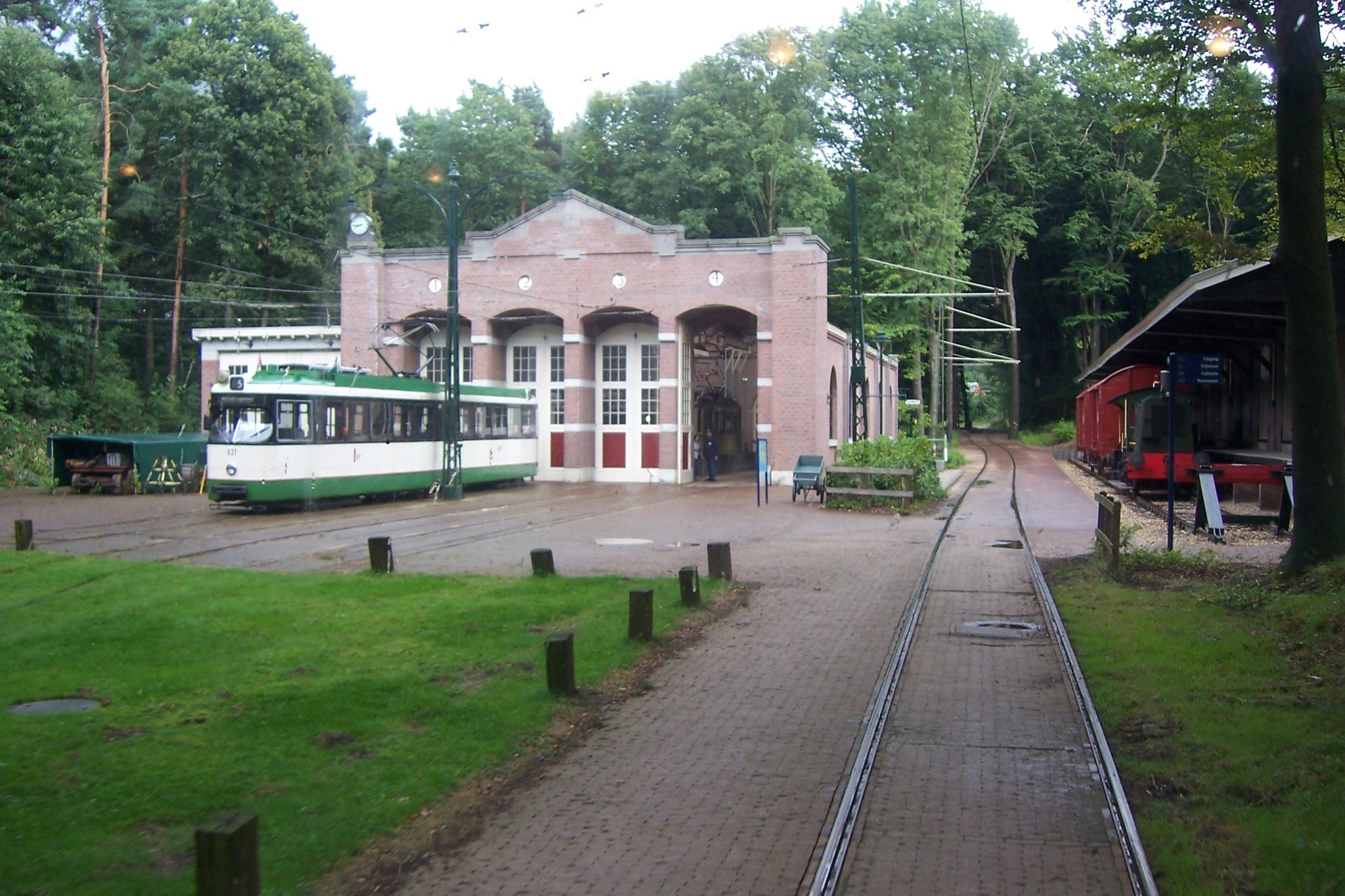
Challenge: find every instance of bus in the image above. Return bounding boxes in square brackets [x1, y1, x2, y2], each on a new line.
[203, 316, 540, 515]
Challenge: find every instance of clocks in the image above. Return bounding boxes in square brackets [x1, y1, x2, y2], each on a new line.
[349, 214, 373, 235]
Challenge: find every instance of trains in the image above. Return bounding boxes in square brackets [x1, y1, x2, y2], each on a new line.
[1074, 361, 1200, 500]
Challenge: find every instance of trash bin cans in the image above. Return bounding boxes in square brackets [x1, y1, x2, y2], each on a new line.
[181, 463, 200, 492]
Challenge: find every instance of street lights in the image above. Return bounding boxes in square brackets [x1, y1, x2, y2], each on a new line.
[343, 153, 572, 504]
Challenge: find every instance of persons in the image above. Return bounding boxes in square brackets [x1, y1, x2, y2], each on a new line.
[691, 431, 702, 482]
[703, 427, 719, 481]
